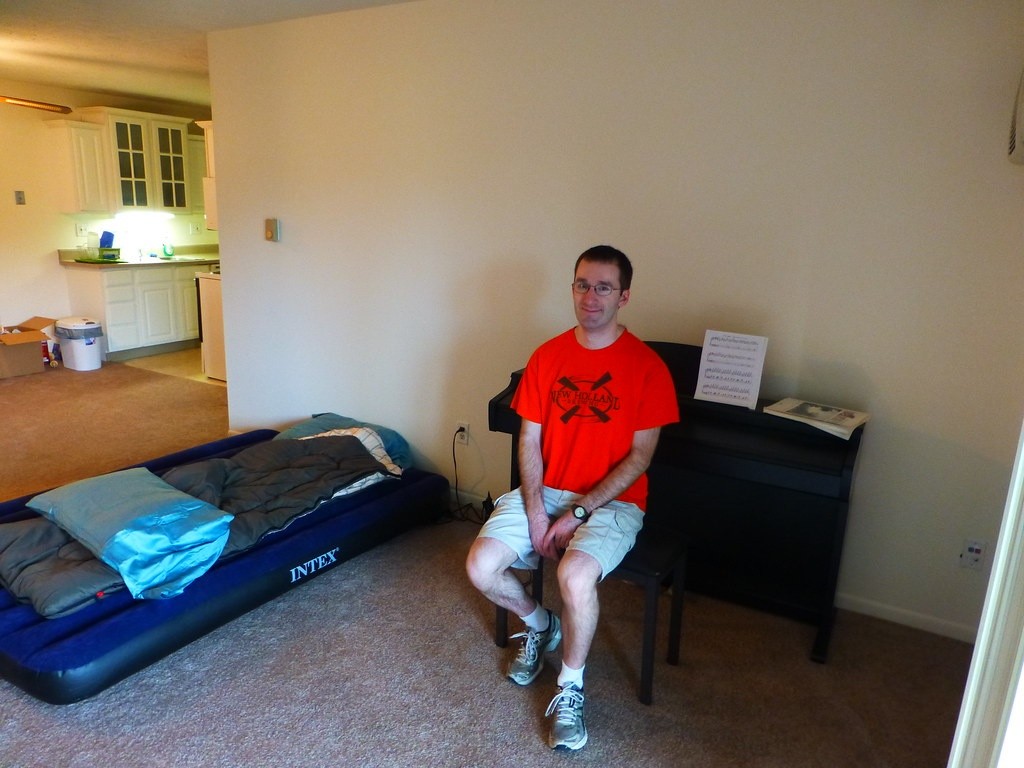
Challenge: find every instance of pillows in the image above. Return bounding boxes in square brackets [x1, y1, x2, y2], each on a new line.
[23, 466, 236, 600]
[273, 413, 414, 470]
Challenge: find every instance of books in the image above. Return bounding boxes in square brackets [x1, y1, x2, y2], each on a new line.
[762, 396, 871, 441]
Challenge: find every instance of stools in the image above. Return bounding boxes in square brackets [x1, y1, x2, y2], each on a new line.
[496, 522, 686, 706]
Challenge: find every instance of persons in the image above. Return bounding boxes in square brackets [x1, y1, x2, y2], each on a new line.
[464, 244, 681, 749]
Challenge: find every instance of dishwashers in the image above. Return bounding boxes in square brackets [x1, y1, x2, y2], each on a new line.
[210, 263, 220, 274]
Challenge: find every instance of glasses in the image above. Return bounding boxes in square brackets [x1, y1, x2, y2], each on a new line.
[571, 281, 623, 296]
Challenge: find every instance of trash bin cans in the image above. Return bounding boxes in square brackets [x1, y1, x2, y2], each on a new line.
[55, 316, 104, 371]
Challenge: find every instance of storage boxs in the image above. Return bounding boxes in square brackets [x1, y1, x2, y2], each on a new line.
[0, 316, 58, 379]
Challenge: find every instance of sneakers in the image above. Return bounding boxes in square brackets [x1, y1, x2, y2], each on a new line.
[545, 680, 588, 750]
[505, 608, 563, 685]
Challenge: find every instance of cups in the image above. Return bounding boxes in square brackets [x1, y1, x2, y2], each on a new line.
[87, 231, 100, 247]
[76, 246, 99, 259]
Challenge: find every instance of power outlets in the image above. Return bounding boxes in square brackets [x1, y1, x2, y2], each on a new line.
[456, 423, 469, 445]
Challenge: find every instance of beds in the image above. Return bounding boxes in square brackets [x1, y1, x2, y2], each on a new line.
[0, 426, 450, 706]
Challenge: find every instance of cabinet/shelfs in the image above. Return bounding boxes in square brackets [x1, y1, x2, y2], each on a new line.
[40, 105, 205, 214]
[66, 264, 226, 381]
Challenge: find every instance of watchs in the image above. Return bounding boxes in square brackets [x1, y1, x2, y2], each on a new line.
[570, 503, 590, 522]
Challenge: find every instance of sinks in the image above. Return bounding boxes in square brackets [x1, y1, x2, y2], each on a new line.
[159, 256, 204, 262]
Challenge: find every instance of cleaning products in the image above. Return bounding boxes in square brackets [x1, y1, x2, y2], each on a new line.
[163, 237, 175, 256]
